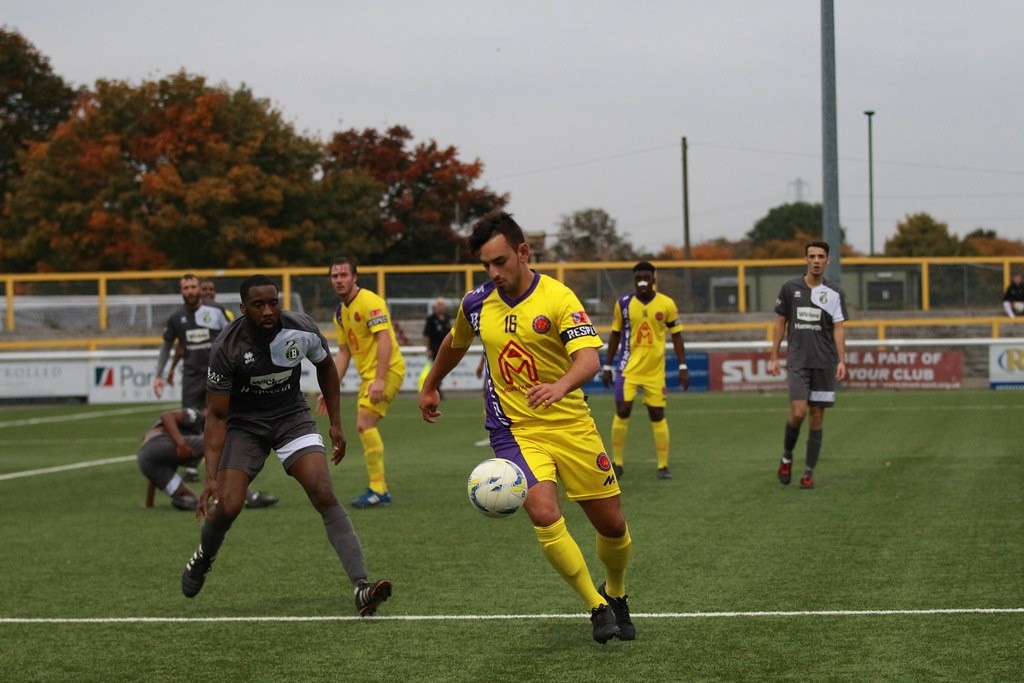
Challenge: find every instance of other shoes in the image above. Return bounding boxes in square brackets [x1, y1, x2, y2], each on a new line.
[171, 497, 197, 510]
[182, 473, 200, 483]
[474, 439, 491, 446]
[246, 490, 279, 509]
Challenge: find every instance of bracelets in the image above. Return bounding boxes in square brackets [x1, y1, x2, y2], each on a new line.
[603, 365, 611, 370]
[679, 364, 687, 370]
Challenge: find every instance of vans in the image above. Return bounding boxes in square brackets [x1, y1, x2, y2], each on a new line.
[385, 295, 465, 347]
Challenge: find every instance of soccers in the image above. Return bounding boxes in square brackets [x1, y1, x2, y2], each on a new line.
[467, 457, 528, 519]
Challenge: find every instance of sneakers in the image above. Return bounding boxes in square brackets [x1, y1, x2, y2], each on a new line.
[182, 543, 218, 597]
[598, 581, 635, 641]
[657, 466, 671, 479]
[611, 462, 623, 479]
[588, 603, 621, 643]
[800, 476, 814, 489]
[352, 490, 391, 507]
[355, 580, 392, 617]
[777, 454, 792, 484]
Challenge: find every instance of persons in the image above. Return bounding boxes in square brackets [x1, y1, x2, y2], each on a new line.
[417, 213, 635, 643]
[476, 357, 485, 378]
[138, 407, 278, 510]
[316, 258, 406, 508]
[422, 300, 453, 399]
[182, 275, 392, 616]
[603, 262, 690, 479]
[153, 273, 235, 482]
[1002, 274, 1024, 318]
[768, 243, 849, 489]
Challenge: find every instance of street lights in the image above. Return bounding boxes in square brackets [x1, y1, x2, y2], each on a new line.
[864, 109, 876, 257]
